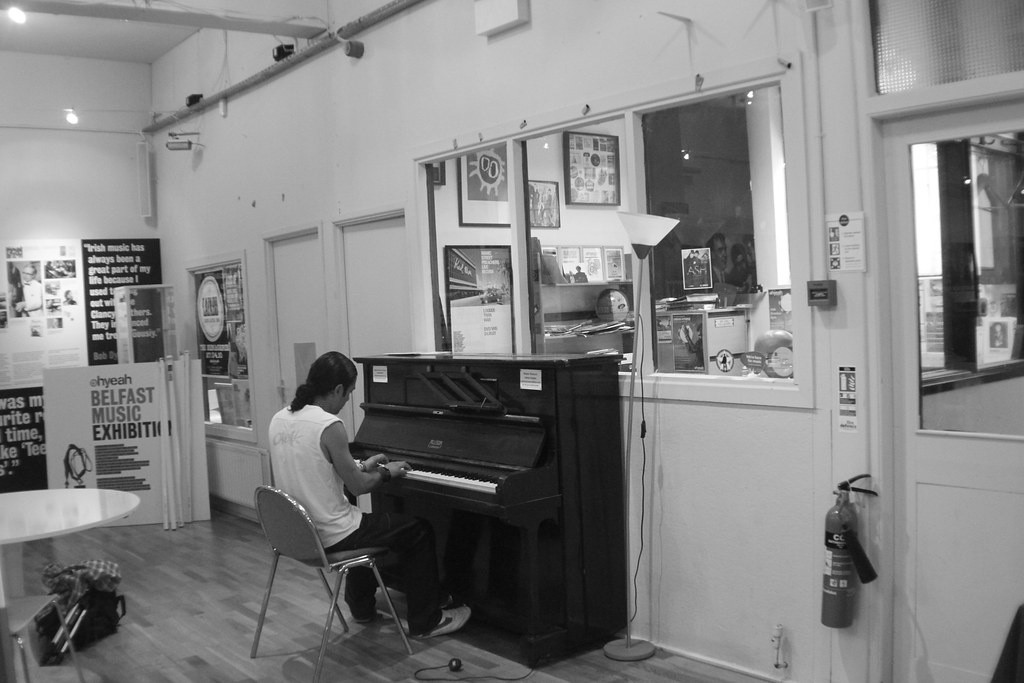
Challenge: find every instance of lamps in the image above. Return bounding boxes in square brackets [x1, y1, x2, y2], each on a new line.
[601, 209, 680, 660]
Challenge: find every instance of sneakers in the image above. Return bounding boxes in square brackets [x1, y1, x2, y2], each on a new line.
[354, 608, 377, 623]
[410, 606, 471, 640]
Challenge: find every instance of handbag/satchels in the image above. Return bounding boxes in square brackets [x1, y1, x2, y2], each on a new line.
[34, 589, 126, 654]
[41, 560, 123, 607]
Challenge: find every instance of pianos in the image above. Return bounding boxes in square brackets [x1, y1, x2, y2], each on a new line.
[341, 350, 627, 664]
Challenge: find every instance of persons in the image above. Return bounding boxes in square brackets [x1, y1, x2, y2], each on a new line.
[683, 324, 704, 369]
[684, 233, 757, 293]
[570, 266, 588, 283]
[8, 261, 77, 328]
[270, 351, 472, 640]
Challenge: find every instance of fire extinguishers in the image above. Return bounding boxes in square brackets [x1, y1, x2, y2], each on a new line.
[820, 474, 878, 627]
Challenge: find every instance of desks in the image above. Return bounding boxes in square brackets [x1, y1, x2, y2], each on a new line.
[0, 486, 141, 683]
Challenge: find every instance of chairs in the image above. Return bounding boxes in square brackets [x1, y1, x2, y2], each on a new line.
[0, 593, 88, 683]
[250, 484, 414, 682]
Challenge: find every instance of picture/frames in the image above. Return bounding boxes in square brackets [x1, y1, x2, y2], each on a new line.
[563, 130, 621, 206]
[542, 245, 626, 282]
[458, 141, 528, 228]
[529, 180, 560, 228]
[431, 160, 446, 185]
[444, 244, 513, 357]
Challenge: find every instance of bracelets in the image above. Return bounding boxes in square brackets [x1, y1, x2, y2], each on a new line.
[357, 461, 366, 472]
[375, 467, 391, 481]
